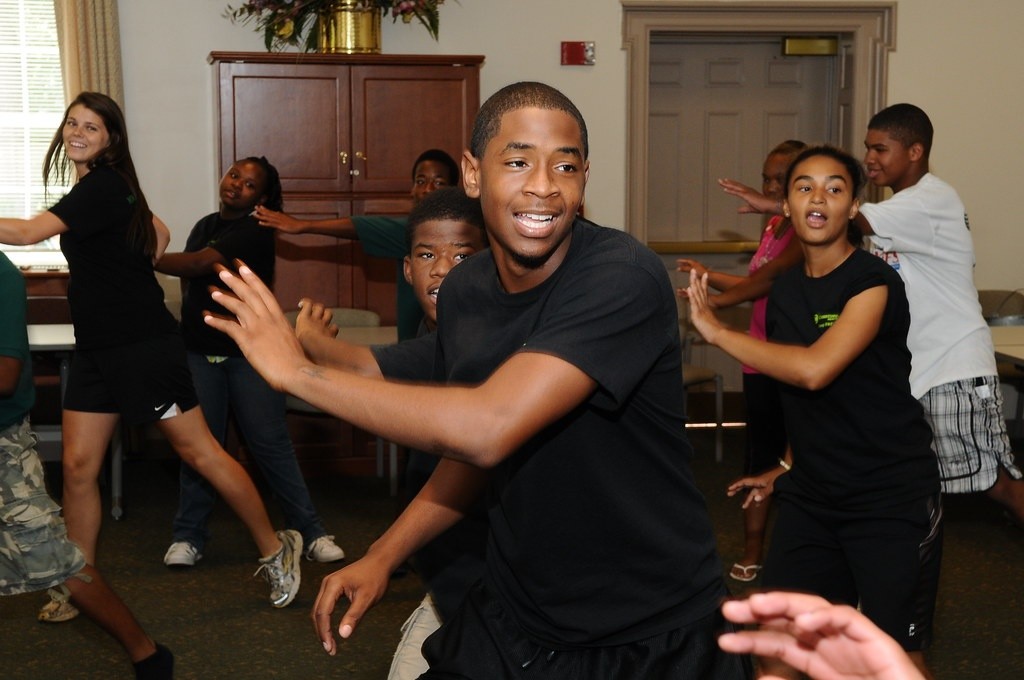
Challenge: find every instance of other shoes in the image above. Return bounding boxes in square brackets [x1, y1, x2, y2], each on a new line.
[133, 642, 174, 680]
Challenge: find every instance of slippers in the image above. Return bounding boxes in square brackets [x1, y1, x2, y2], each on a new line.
[730, 563, 762, 581]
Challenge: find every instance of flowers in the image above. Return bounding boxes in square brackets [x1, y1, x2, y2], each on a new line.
[222, 0, 464, 73]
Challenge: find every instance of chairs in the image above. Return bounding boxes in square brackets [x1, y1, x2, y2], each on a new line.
[283, 309, 386, 477]
[681, 363, 724, 463]
[978, 290, 1024, 440]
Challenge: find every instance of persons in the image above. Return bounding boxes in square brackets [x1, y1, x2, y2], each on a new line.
[685, 144, 945, 680]
[719, 590, 925, 678]
[0, 248, 175, 678]
[674, 138, 814, 580]
[718, 104, 1023, 522]
[1, 92, 303, 623]
[202, 78, 750, 680]
[152, 155, 347, 563]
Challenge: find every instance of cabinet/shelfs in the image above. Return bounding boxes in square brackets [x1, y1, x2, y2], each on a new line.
[206, 51, 485, 480]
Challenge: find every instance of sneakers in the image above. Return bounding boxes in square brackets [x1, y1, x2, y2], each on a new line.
[303, 535, 345, 562]
[163, 542, 202, 566]
[253, 530, 304, 608]
[39, 599, 80, 622]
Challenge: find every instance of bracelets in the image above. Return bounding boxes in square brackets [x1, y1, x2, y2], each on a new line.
[778, 457, 792, 471]
[776, 199, 781, 216]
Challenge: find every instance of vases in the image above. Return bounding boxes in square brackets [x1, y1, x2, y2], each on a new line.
[317, 0, 381, 54]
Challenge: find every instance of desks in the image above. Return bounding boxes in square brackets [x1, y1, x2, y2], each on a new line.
[24, 324, 397, 520]
[990, 325, 1024, 367]
